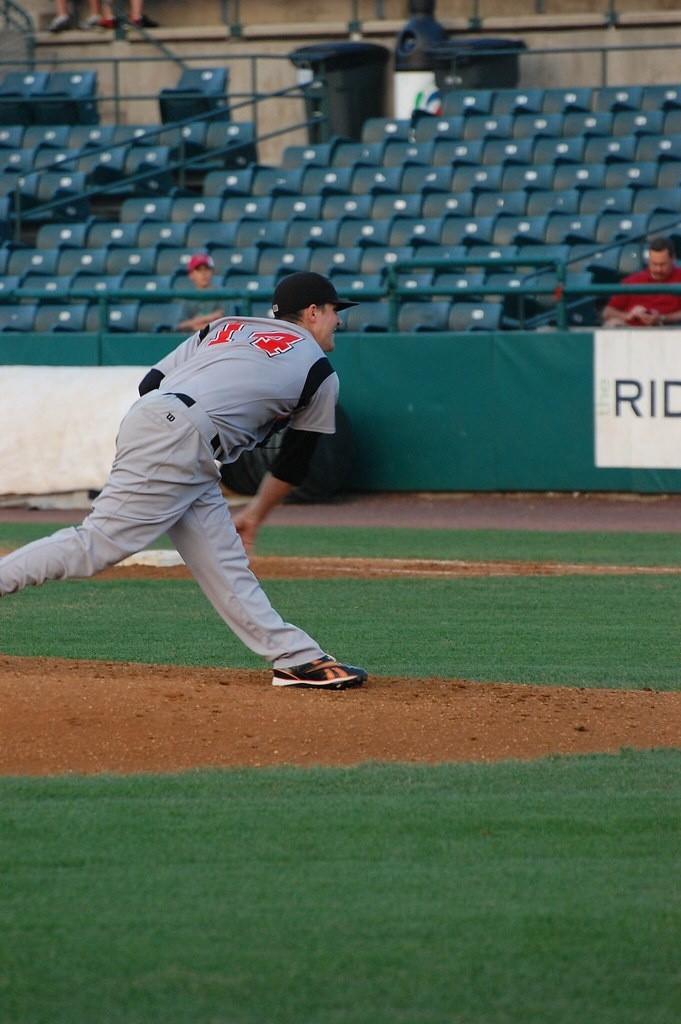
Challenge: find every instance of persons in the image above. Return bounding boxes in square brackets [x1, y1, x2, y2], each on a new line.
[1, 273, 368, 687]
[602, 238, 681, 327]
[47, 0, 159, 30]
[174, 253, 225, 332]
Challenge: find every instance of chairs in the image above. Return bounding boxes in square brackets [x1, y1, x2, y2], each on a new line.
[0, 68, 681, 335]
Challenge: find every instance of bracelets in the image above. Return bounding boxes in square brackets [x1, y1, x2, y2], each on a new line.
[660, 315, 664, 321]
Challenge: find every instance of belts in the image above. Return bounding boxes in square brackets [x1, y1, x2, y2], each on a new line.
[163, 393, 226, 463]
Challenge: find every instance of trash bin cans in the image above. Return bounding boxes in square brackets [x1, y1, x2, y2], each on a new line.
[421, 35, 528, 91]
[159, 66, 233, 124]
[0, 71, 102, 125]
[287, 32, 390, 146]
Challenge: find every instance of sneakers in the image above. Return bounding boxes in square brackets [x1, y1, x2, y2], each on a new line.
[271, 654, 368, 689]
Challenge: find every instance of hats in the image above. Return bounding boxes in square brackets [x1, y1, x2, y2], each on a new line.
[272, 272, 359, 316]
[189, 254, 215, 270]
